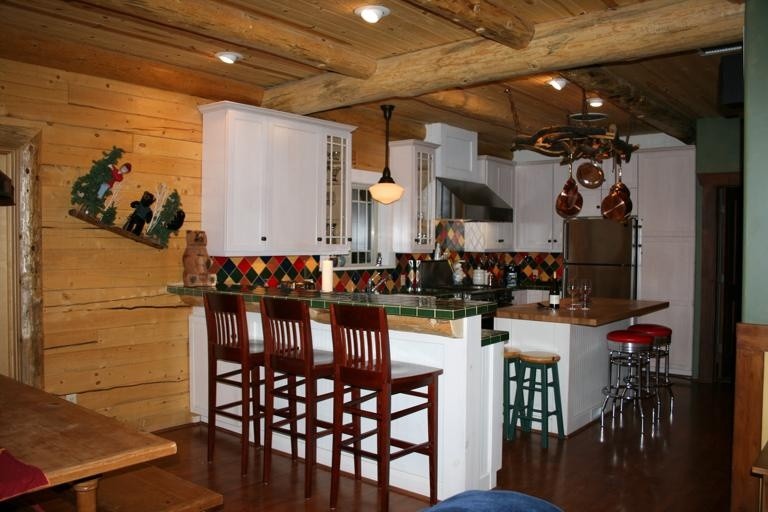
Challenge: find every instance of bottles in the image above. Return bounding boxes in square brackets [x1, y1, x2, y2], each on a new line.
[485, 271, 488, 285]
[435, 243, 441, 261]
[549, 269, 560, 310]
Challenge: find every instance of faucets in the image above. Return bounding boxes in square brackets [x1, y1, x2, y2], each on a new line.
[367, 272, 393, 293]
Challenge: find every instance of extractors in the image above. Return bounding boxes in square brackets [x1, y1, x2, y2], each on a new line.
[436, 177, 514, 224]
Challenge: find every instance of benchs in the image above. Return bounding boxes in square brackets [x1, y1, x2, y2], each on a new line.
[96, 463, 223, 511]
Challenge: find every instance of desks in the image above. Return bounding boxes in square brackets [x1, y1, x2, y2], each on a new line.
[0, 375, 178, 512]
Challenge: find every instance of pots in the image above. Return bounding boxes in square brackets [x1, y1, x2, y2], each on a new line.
[555, 150, 632, 224]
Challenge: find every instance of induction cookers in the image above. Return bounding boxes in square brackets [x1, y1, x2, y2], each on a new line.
[442, 285, 511, 293]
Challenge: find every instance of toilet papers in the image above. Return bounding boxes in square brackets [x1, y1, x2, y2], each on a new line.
[322, 260, 333, 293]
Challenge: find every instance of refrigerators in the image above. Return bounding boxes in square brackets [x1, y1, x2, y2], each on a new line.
[563, 216, 642, 302]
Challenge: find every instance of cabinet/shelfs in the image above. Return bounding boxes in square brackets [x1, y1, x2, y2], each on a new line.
[462, 161, 514, 252]
[514, 155, 638, 252]
[198, 101, 353, 255]
[388, 139, 438, 254]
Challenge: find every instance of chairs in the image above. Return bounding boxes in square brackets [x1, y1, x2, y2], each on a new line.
[329, 302, 443, 512]
[259, 296, 363, 499]
[202, 291, 299, 478]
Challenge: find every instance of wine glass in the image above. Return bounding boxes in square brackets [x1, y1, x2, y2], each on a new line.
[580, 278, 592, 310]
[566, 278, 579, 311]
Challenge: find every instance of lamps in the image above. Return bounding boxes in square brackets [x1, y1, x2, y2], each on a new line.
[545, 77, 570, 90]
[214, 52, 242, 64]
[586, 97, 606, 107]
[354, 4, 390, 24]
[369, 104, 404, 204]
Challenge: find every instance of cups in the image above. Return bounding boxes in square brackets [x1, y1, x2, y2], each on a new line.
[453, 289, 471, 303]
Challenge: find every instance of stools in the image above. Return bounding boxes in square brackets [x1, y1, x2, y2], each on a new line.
[507, 351, 565, 449]
[504, 347, 526, 435]
[599, 330, 656, 449]
[628, 323, 675, 421]
